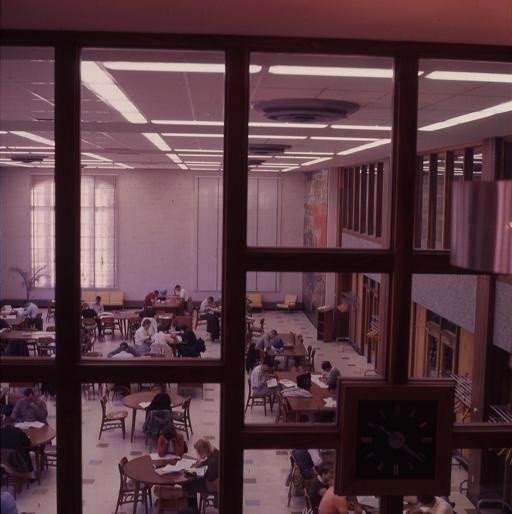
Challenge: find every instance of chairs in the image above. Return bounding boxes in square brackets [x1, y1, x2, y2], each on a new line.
[0, 291, 316, 514]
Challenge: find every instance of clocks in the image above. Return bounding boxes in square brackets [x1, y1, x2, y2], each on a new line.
[335, 377, 456, 497]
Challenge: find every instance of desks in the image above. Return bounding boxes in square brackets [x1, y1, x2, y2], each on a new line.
[276, 370, 337, 424]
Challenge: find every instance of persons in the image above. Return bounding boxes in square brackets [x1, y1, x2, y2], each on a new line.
[250, 329, 342, 403]
[1, 384, 49, 513]
[293, 447, 452, 513]
[1, 300, 38, 328]
[146, 385, 219, 512]
[82, 285, 215, 358]
